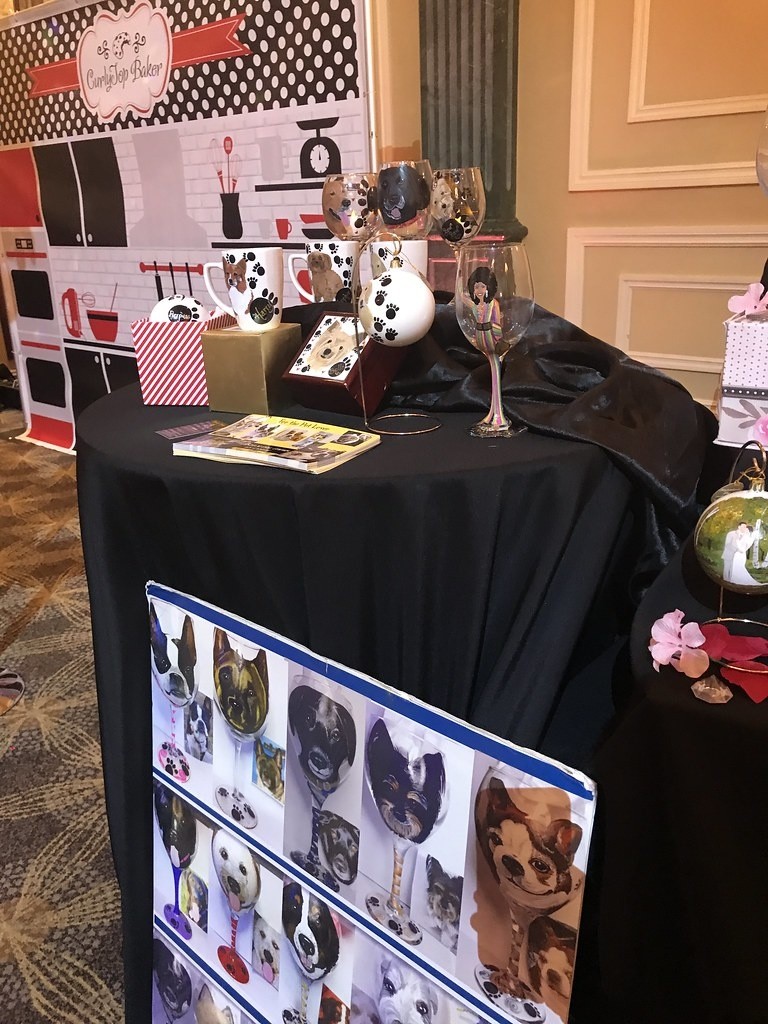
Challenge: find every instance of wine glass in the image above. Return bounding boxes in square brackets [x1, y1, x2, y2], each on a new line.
[456, 245, 534, 438]
[145, 594, 592, 1024]
[430, 167, 485, 305]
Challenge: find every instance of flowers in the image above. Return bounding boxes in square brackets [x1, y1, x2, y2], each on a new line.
[648, 608, 710, 680]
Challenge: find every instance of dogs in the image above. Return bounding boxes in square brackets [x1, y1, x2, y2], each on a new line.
[145, 601, 581, 1023]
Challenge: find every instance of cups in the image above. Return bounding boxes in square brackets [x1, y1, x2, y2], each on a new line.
[379, 159, 434, 240]
[202, 247, 283, 332]
[321, 172, 379, 241]
[370, 240, 427, 281]
[289, 241, 361, 302]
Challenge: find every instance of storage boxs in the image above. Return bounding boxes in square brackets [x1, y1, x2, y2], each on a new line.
[281, 311, 396, 419]
[713, 308, 768, 452]
[200, 322, 303, 416]
[131, 309, 244, 407]
